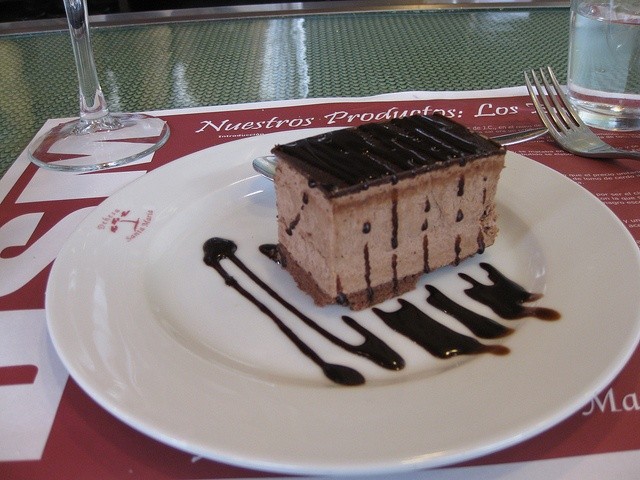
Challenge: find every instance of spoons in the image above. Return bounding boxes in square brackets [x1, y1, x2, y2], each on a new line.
[252, 128, 547, 182]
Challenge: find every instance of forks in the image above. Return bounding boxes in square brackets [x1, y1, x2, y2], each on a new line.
[522, 64, 640, 163]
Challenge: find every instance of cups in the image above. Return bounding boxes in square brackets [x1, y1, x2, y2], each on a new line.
[566, 1, 639, 133]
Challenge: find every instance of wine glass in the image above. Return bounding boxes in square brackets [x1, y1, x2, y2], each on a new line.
[28, 0, 170, 172]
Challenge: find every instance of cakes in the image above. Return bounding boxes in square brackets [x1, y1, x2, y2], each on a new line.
[261, 110, 508, 311]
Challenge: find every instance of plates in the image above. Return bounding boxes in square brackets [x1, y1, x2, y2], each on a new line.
[42, 124, 639, 475]
